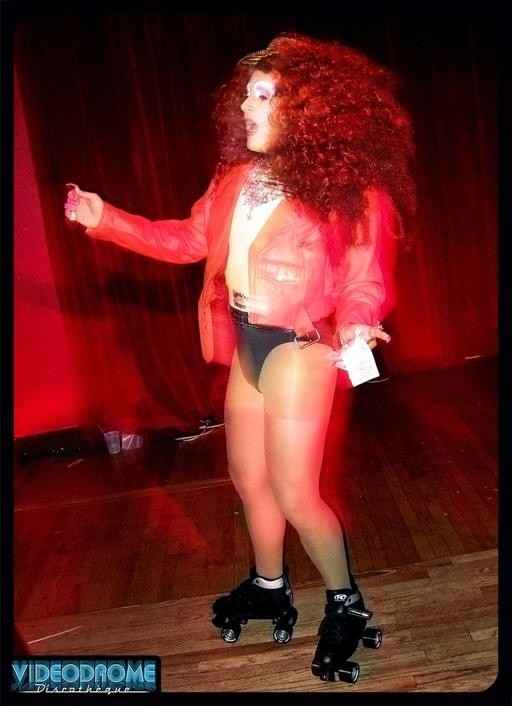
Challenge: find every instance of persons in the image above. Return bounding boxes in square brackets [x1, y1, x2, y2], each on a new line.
[64, 34, 400, 674]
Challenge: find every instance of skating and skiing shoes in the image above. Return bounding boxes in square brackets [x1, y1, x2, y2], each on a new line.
[212, 565, 298, 644]
[311, 582, 382, 683]
